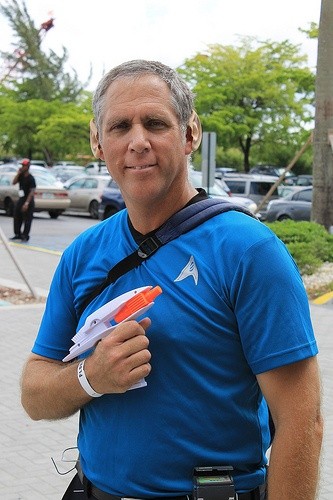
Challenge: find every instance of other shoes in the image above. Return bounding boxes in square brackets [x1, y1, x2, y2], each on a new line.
[10, 236, 28, 242]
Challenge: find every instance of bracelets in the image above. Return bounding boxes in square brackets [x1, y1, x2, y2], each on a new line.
[24, 202, 29, 205]
[77, 358, 104, 397]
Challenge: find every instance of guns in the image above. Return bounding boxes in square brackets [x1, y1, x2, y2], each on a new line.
[62, 284, 163, 389]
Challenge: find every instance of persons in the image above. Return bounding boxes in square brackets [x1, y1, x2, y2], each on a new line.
[8, 158, 36, 243]
[18, 59, 324, 500]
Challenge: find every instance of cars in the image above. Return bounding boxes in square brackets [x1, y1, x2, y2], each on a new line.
[63, 172, 113, 220]
[1, 163, 73, 220]
[0, 157, 315, 223]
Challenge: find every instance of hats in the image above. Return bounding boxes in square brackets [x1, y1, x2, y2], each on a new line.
[18, 159, 30, 165]
